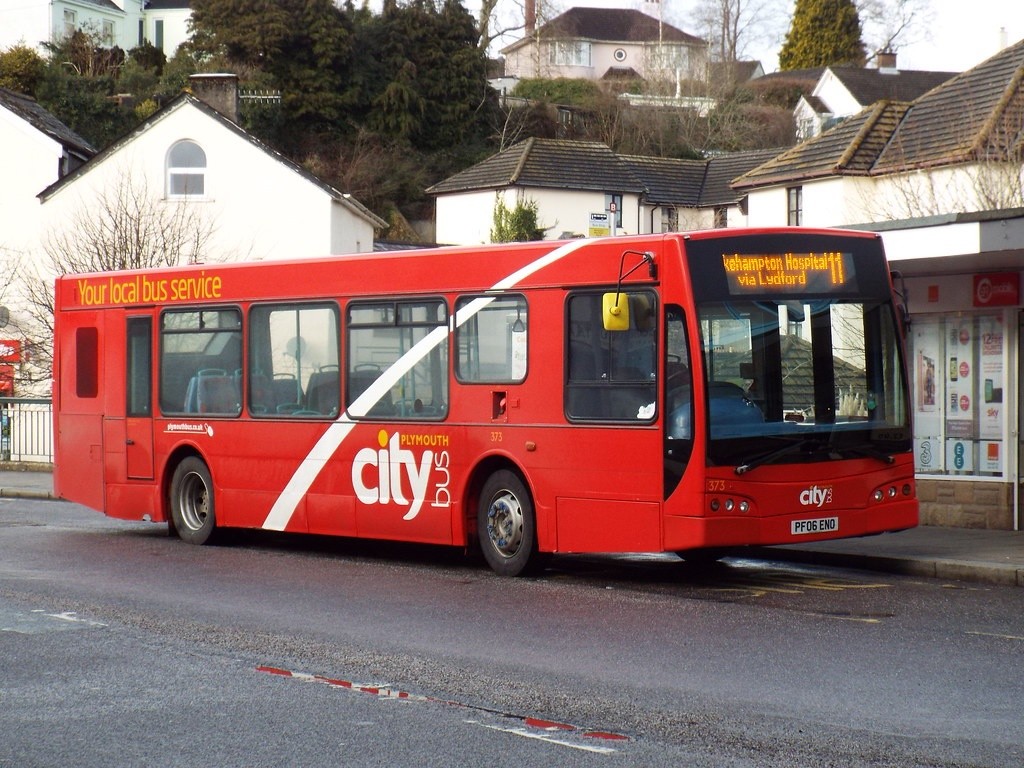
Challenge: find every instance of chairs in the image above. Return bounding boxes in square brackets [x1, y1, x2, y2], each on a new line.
[663, 352, 689, 387]
[177, 361, 434, 422]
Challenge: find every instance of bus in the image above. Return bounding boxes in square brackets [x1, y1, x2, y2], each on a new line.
[52, 226, 923, 579]
